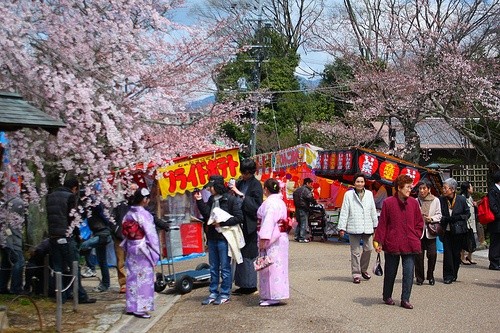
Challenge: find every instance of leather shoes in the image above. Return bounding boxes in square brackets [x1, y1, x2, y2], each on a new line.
[401, 299, 413, 309]
[385, 298, 395, 305]
[354, 278, 360, 283]
[362, 271, 371, 279]
[79, 297, 97, 304]
[417, 277, 456, 286]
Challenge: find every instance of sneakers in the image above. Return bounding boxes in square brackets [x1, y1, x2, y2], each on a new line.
[203, 294, 229, 305]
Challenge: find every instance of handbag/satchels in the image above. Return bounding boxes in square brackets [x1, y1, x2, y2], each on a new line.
[373, 252, 383, 276]
[460, 215, 476, 253]
[427, 221, 444, 236]
[253, 246, 273, 272]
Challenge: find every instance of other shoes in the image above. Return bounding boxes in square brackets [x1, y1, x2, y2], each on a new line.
[120, 287, 126, 292]
[259, 299, 279, 306]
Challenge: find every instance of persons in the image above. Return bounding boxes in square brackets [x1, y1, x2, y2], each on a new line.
[487, 172, 500, 271]
[110, 196, 171, 243]
[46, 179, 97, 305]
[374, 175, 425, 308]
[412, 178, 442, 286]
[119, 184, 160, 318]
[291, 177, 320, 243]
[253, 177, 295, 306]
[78, 181, 113, 292]
[370, 182, 387, 210]
[1, 180, 30, 295]
[458, 181, 478, 264]
[79, 218, 96, 278]
[227, 159, 262, 296]
[193, 175, 246, 306]
[199, 185, 212, 246]
[439, 178, 471, 284]
[336, 174, 379, 283]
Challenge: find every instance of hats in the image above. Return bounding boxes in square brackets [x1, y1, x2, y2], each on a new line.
[203, 176, 223, 188]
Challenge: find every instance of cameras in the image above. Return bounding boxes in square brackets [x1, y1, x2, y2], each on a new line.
[194, 188, 200, 192]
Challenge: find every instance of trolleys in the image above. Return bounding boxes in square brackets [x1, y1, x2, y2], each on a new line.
[155, 221, 211, 293]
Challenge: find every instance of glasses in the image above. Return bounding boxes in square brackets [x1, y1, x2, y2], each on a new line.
[442, 186, 451, 190]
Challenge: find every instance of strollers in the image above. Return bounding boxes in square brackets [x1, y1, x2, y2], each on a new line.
[305, 203, 328, 241]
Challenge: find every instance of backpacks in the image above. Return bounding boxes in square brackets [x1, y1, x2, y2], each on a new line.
[477, 188, 496, 226]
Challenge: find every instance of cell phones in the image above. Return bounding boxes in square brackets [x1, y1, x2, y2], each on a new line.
[229, 178, 234, 187]
